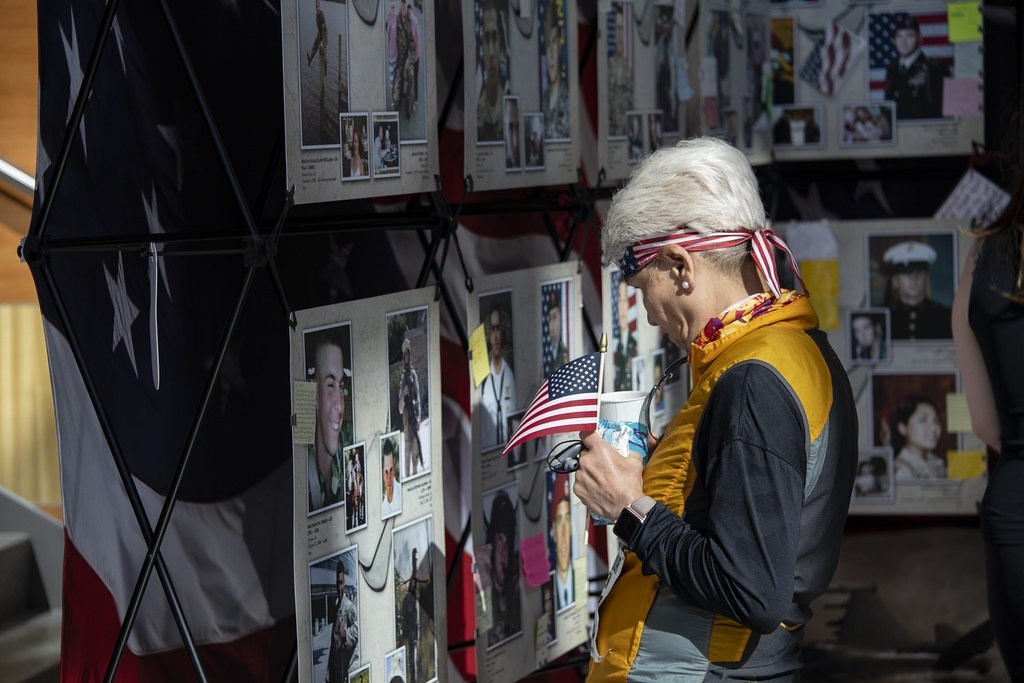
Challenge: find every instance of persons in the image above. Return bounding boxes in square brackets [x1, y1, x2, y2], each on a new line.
[305, 2, 944, 179]
[853, 243, 950, 493]
[572, 136, 857, 683]
[306, 282, 664, 683]
[952, 162, 1024, 680]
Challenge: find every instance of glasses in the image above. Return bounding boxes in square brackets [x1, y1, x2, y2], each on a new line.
[638, 355, 687, 441]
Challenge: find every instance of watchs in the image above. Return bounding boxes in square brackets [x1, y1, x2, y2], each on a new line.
[612, 495, 657, 546]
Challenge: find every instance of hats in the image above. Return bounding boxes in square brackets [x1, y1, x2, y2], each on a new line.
[545, 292, 559, 307]
[486, 492, 514, 556]
[897, 17, 919, 31]
[883, 241, 938, 271]
[550, 470, 570, 521]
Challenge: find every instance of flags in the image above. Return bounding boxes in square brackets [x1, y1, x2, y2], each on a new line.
[866, 11, 954, 90]
[500, 352, 601, 457]
[796, 19, 865, 99]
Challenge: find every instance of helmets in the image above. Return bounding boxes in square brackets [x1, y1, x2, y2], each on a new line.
[402, 339, 412, 353]
